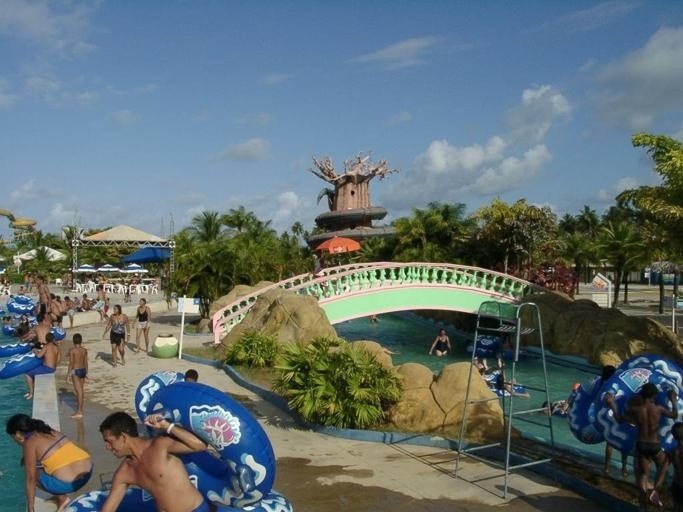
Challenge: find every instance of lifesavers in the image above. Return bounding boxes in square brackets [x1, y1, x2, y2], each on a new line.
[468, 334, 524, 361]
[0, 295, 65, 379]
[483, 370, 524, 397]
[61, 372, 293, 512]
[549, 355, 683, 456]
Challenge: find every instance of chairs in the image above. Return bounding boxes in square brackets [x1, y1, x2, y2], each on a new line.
[56, 278, 63, 285]
[76, 281, 158, 295]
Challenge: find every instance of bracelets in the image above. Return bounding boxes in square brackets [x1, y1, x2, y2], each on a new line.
[166, 423, 176, 435]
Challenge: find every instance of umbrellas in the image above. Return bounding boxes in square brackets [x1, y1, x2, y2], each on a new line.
[71, 264, 98, 284]
[313, 235, 362, 270]
[98, 264, 122, 278]
[119, 263, 150, 275]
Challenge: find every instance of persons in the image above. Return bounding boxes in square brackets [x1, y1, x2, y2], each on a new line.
[607, 382, 678, 512]
[99, 412, 213, 511]
[103, 304, 131, 368]
[66, 333, 90, 418]
[428, 329, 452, 357]
[312, 254, 326, 289]
[2, 271, 151, 354]
[380, 345, 401, 357]
[664, 422, 682, 510]
[6, 414, 95, 512]
[25, 332, 59, 401]
[573, 365, 628, 479]
[482, 358, 530, 397]
[184, 369, 199, 384]
[472, 356, 484, 370]
[370, 315, 382, 325]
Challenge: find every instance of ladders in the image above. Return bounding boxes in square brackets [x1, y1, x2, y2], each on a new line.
[454, 300, 559, 498]
